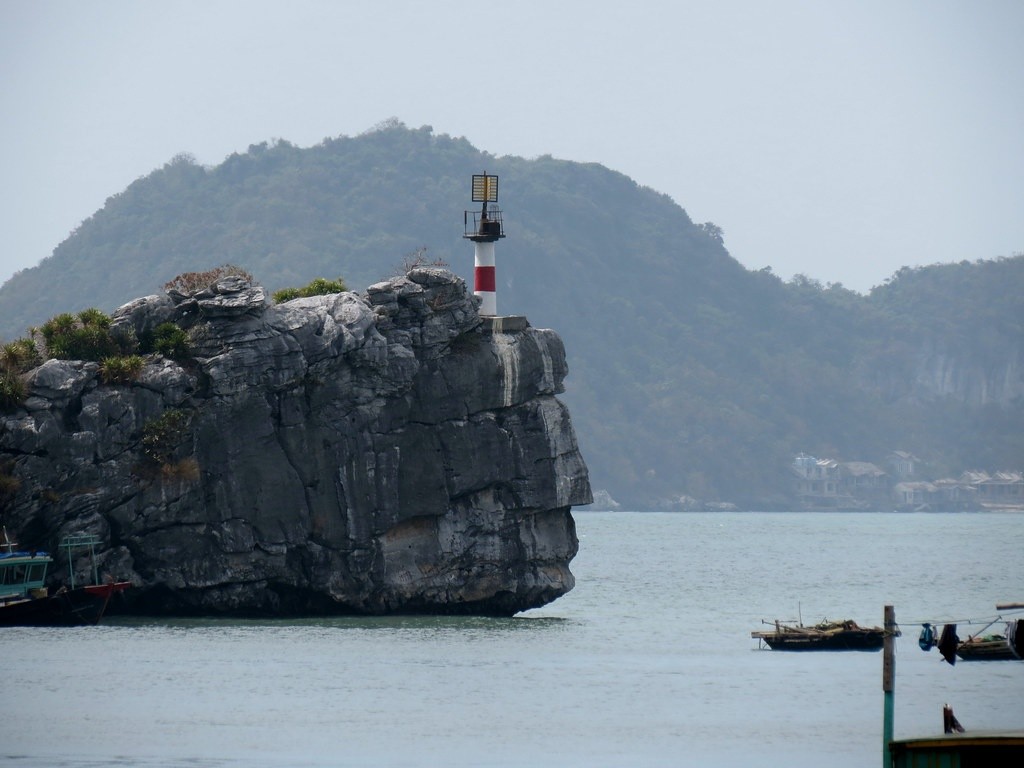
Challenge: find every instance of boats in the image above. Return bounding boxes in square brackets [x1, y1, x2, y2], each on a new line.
[0, 525, 130, 627]
[751, 619, 884, 651]
[955, 636, 1017, 661]
